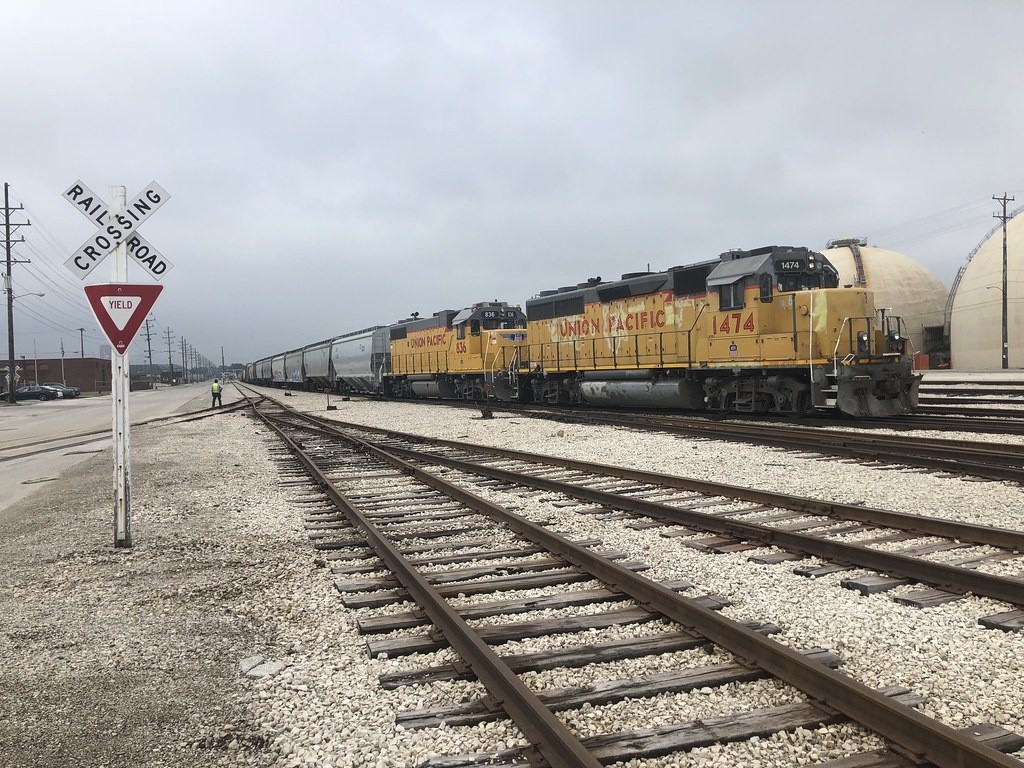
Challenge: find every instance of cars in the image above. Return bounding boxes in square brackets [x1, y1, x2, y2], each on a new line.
[0, 382, 80, 401]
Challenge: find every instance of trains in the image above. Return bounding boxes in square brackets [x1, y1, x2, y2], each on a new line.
[236, 244, 932, 424]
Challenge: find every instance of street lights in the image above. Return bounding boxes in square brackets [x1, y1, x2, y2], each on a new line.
[7, 292, 46, 405]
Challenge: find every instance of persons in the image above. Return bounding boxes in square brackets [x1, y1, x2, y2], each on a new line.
[211, 380, 223, 408]
